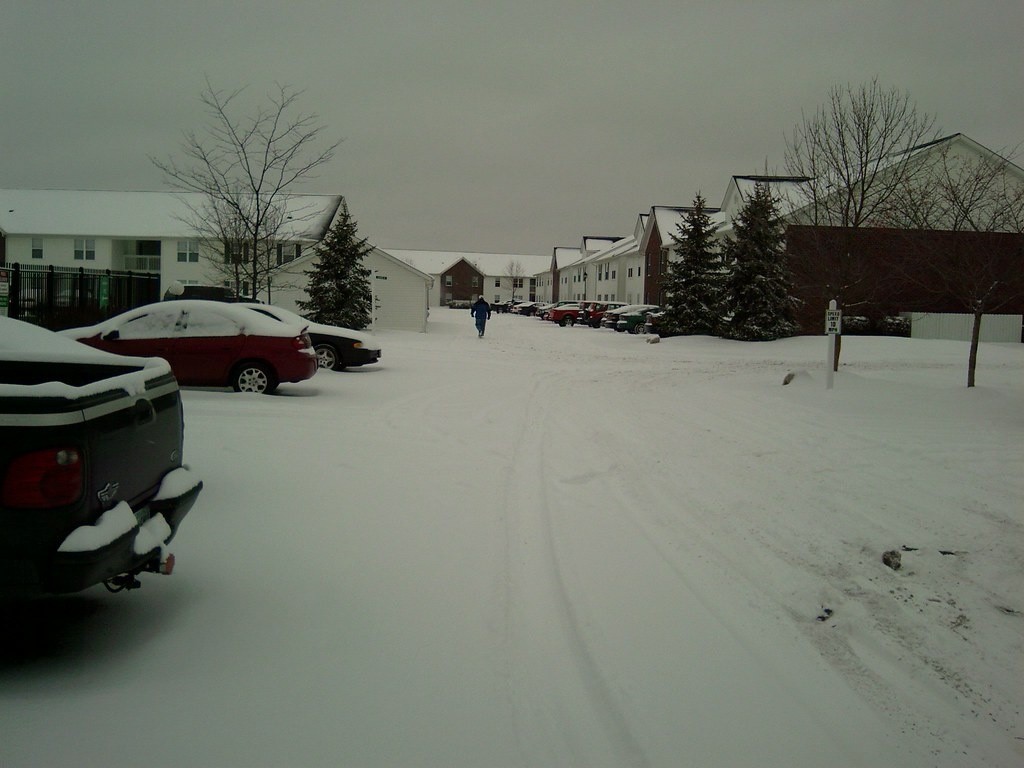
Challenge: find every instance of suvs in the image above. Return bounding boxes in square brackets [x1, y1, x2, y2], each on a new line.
[163, 281, 265, 303]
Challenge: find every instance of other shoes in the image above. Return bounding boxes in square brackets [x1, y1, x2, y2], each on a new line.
[479, 330, 484, 336]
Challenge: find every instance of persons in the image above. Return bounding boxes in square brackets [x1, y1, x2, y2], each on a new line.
[470, 295, 491, 337]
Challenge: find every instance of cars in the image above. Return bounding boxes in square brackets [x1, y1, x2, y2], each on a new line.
[233, 302, 383, 371]
[0, 314, 206, 599]
[490, 297, 667, 336]
[55, 300, 318, 396]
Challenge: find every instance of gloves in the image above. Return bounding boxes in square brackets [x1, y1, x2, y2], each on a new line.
[471, 313, 474, 317]
[487, 316, 490, 320]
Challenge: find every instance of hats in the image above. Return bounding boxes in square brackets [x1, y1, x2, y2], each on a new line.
[479, 295, 484, 299]
